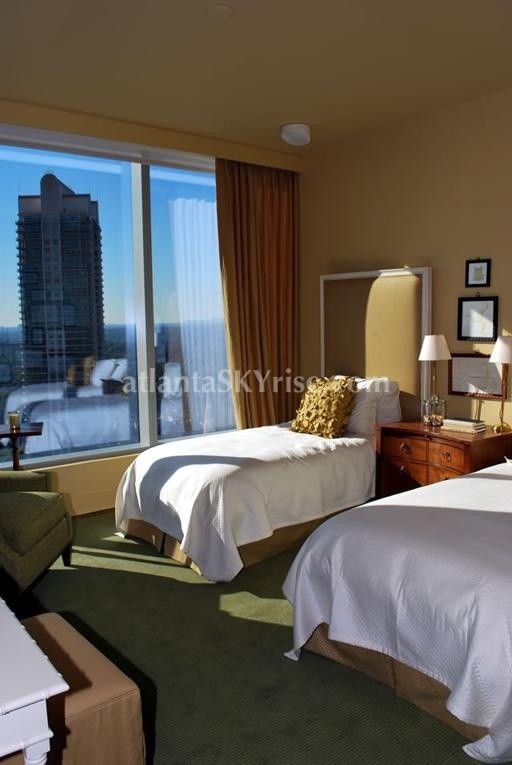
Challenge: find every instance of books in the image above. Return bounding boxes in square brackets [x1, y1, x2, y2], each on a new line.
[441, 416, 487, 434]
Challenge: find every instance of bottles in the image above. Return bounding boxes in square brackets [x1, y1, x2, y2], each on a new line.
[421, 394, 446, 427]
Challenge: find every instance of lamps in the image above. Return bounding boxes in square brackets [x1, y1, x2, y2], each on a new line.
[418, 334, 452, 396]
[489, 336, 512, 432]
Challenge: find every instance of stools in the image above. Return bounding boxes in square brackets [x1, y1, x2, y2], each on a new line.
[0, 612, 146, 765]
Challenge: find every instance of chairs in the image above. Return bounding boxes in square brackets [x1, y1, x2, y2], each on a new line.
[0, 467, 74, 612]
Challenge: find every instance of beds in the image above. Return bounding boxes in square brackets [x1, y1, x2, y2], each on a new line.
[0, 422, 43, 470]
[0, 323, 191, 454]
[115, 267, 433, 583]
[282, 456, 512, 765]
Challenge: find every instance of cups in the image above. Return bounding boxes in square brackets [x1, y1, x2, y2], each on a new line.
[8, 409, 21, 430]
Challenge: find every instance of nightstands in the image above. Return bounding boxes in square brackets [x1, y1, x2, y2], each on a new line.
[373, 417, 512, 498]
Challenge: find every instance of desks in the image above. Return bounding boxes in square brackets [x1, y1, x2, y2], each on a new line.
[0, 596, 70, 765]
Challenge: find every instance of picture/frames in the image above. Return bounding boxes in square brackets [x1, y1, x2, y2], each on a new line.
[448, 352, 508, 400]
[457, 296, 498, 342]
[465, 258, 491, 288]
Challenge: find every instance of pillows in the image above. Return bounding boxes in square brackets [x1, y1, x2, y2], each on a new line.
[289, 374, 403, 439]
